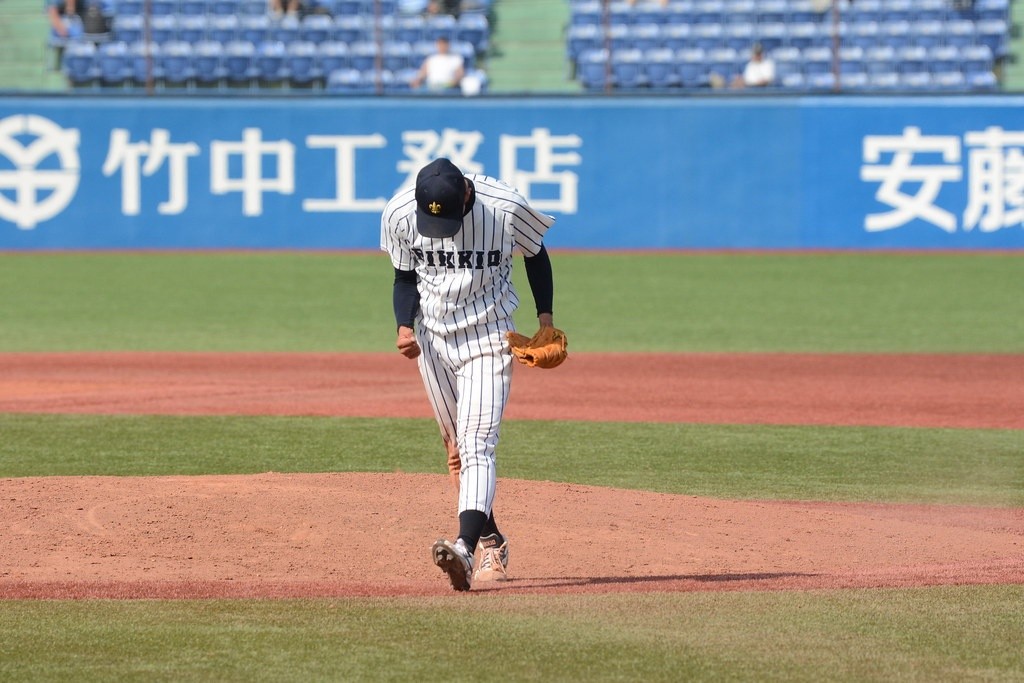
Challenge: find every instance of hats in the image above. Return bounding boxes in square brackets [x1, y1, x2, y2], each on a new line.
[415, 158, 466, 238]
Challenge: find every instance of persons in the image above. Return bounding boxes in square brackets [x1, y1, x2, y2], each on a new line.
[733, 40, 775, 88]
[47, 0, 86, 37]
[270, 0, 301, 14]
[379, 158, 567, 592]
[424, 0, 477, 19]
[409, 38, 466, 89]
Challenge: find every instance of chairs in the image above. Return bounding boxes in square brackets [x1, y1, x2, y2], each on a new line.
[45, 0, 1023, 101]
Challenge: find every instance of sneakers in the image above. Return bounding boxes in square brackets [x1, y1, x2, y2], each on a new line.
[475, 533, 509, 581]
[432, 539, 475, 591]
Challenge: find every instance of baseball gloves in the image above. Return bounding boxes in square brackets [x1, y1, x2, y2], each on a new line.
[505, 322, 573, 370]
